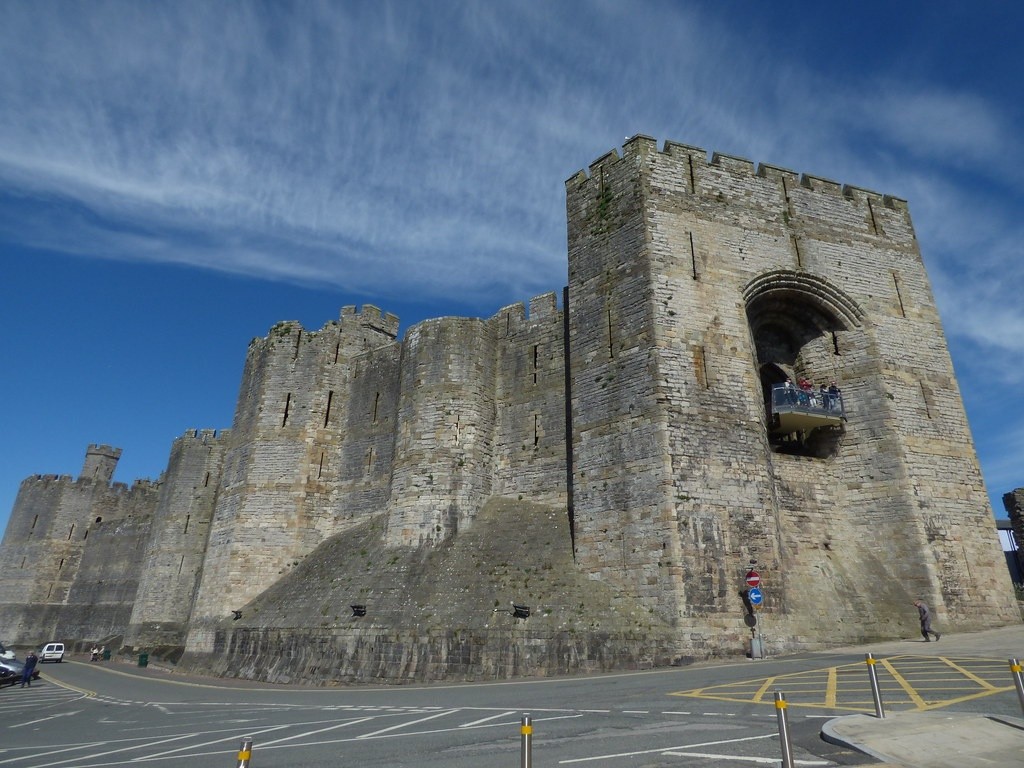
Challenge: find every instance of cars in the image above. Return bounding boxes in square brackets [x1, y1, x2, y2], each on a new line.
[0, 644, 40, 682]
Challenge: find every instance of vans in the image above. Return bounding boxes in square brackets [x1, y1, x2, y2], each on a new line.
[37, 643, 65, 664]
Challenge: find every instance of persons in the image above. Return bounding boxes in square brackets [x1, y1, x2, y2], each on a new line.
[783, 376, 841, 411]
[90, 643, 104, 662]
[20, 651, 38, 688]
[914, 600, 941, 642]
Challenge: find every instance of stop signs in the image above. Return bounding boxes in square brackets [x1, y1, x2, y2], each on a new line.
[745, 571, 760, 587]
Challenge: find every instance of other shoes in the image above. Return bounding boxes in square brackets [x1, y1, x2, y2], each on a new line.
[936, 635, 940, 641]
[28, 685, 30, 687]
[21, 685, 24, 688]
[924, 639, 930, 642]
